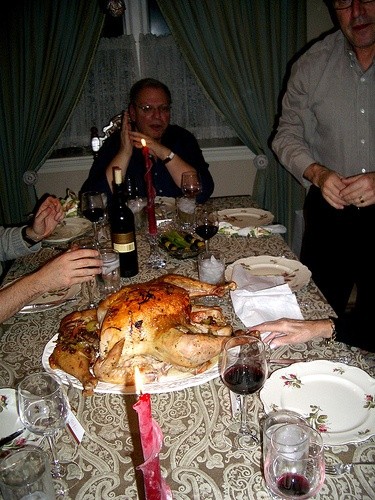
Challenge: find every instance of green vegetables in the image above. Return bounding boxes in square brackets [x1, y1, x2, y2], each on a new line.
[164, 227, 190, 248]
[58, 187, 80, 217]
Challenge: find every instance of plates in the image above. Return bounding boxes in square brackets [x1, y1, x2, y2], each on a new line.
[45, 217, 93, 242]
[125, 196, 177, 218]
[260, 360, 375, 446]
[0, 388, 44, 471]
[0, 275, 82, 314]
[224, 254, 312, 293]
[209, 208, 275, 226]
[42, 332, 239, 396]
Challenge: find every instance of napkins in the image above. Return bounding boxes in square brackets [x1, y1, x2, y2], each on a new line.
[219, 222, 288, 237]
[229, 283, 305, 334]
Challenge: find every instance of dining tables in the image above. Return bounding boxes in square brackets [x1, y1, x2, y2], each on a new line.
[0, 194, 375, 500]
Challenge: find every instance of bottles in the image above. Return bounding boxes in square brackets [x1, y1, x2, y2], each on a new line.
[107, 168, 140, 277]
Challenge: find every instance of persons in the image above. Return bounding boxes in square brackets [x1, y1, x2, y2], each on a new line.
[0, 197, 102, 323]
[270, 0, 375, 314]
[80, 77, 215, 209]
[250, 312, 375, 354]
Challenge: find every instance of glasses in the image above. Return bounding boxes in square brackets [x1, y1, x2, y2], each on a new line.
[332, 0, 375, 10]
[136, 105, 170, 113]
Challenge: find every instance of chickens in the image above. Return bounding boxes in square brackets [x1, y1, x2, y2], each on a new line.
[94, 273, 262, 384]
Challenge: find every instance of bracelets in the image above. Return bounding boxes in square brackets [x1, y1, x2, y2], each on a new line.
[21, 226, 37, 246]
[325, 318, 337, 343]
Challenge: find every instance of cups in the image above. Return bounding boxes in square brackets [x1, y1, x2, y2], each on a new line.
[197, 251, 225, 286]
[128, 196, 146, 227]
[180, 171, 202, 199]
[263, 411, 308, 488]
[176, 201, 194, 228]
[264, 423, 325, 500]
[0, 447, 55, 500]
[98, 249, 121, 294]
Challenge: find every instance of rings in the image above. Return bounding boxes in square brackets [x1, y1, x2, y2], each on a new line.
[359, 196, 364, 203]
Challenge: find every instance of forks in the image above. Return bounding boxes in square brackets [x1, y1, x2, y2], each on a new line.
[325, 462, 375, 477]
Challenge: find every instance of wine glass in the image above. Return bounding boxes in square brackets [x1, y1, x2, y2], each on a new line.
[81, 192, 105, 246]
[72, 237, 101, 310]
[220, 335, 268, 450]
[18, 372, 81, 494]
[193, 203, 218, 253]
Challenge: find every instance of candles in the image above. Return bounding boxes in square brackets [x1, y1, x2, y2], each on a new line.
[140, 139, 157, 233]
[133, 387, 172, 500]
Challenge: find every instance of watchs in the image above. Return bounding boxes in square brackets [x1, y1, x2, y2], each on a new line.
[160, 151, 175, 164]
